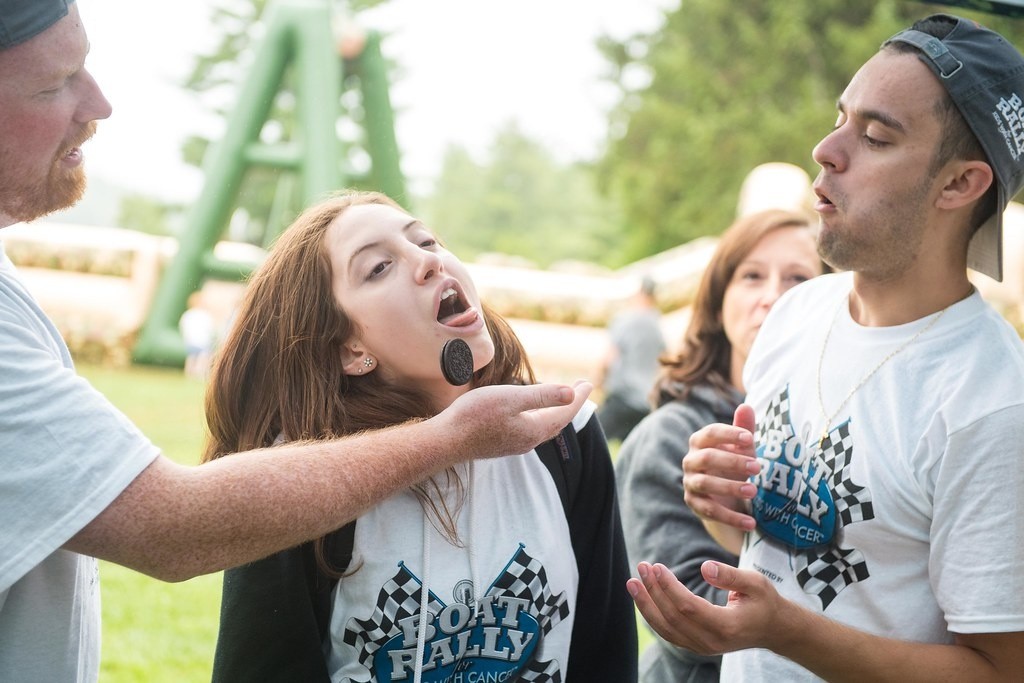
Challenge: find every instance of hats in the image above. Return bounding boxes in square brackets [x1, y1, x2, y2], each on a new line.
[879, 13, 1023, 283]
[0, 0, 76, 54]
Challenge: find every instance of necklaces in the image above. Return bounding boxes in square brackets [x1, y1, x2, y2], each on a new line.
[817, 283, 975, 447]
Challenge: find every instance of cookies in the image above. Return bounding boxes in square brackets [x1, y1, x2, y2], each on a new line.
[439, 338, 474, 386]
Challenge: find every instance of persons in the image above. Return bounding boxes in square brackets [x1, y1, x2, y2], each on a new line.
[0, 0, 592, 683]
[626, 13, 1024, 683]
[179, 292, 211, 373]
[203, 191, 639, 683]
[592, 279, 668, 442]
[609, 209, 835, 683]
